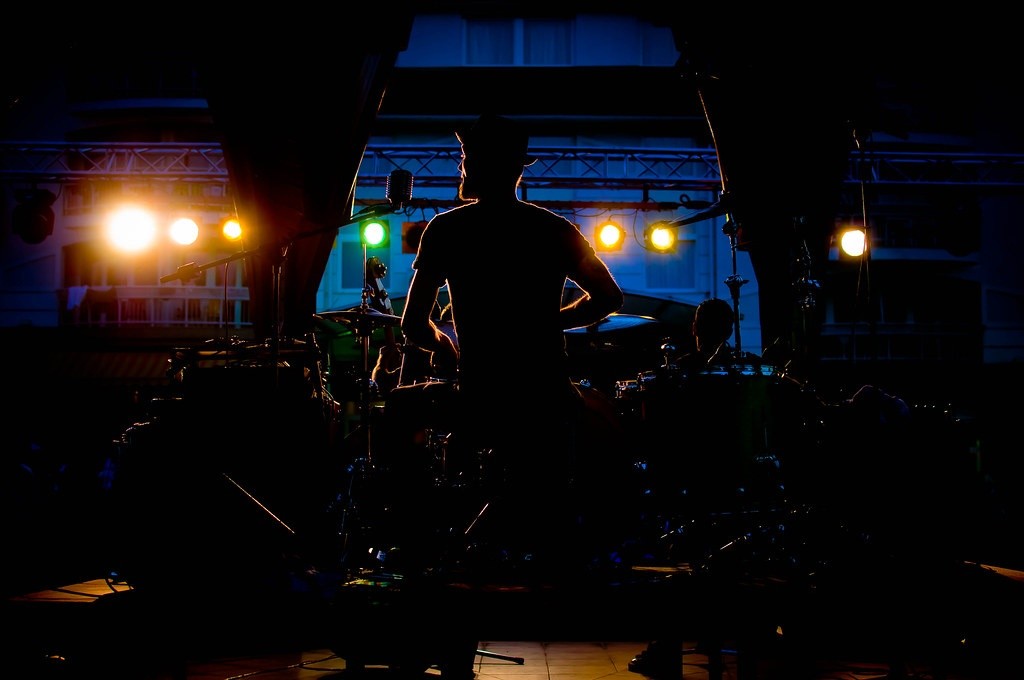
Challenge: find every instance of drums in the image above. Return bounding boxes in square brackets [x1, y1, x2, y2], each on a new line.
[572, 376, 627, 521]
[563, 310, 662, 384]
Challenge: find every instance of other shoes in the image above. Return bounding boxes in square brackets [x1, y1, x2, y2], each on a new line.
[628, 642, 683, 678]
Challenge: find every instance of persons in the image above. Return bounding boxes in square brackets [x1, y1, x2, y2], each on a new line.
[403, 108, 624, 553]
[625, 294, 873, 680]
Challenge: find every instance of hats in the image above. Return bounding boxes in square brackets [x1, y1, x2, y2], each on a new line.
[455, 116, 536, 165]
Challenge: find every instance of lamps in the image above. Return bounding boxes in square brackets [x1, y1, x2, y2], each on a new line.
[360, 219, 390, 249]
[401, 222, 428, 253]
[644, 221, 677, 253]
[594, 221, 627, 253]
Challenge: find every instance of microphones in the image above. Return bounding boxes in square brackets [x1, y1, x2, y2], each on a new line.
[705, 342, 726, 372]
[763, 337, 785, 356]
[360, 169, 414, 215]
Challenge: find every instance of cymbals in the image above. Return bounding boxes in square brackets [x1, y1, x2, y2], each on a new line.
[317, 306, 404, 328]
